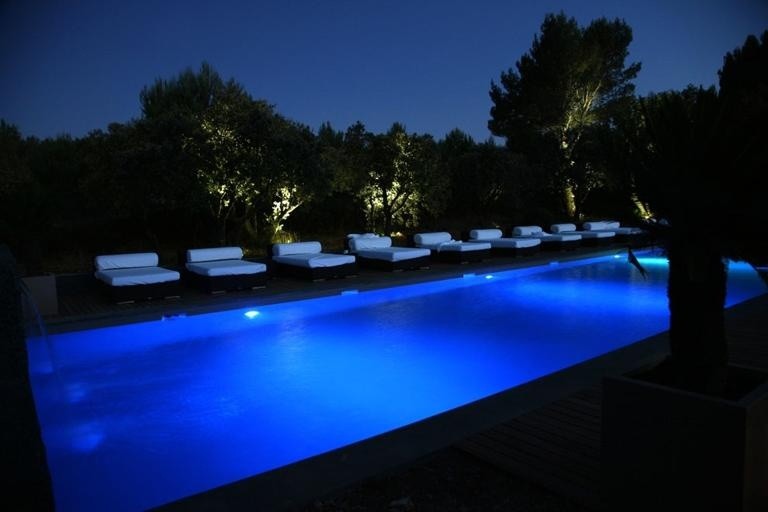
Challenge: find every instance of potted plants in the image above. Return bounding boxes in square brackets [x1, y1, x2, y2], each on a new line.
[600, 88, 768, 512]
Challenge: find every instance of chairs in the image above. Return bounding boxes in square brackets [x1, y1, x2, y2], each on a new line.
[267, 241, 358, 283]
[415, 232, 493, 266]
[96, 252, 183, 305]
[179, 246, 272, 295]
[468, 229, 543, 260]
[549, 224, 616, 250]
[583, 221, 642, 250]
[343, 232, 432, 278]
[511, 226, 582, 254]
